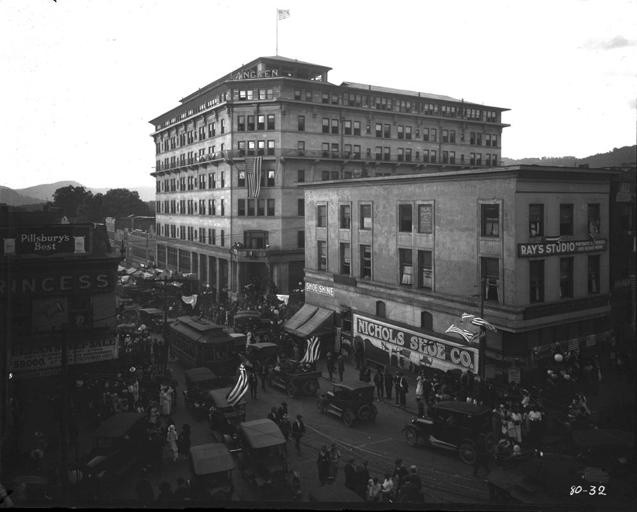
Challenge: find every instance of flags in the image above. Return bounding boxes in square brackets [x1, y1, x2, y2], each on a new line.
[278, 7, 290, 21]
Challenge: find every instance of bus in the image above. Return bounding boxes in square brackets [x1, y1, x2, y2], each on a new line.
[164, 313, 239, 378]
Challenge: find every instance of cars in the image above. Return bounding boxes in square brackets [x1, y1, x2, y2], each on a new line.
[139, 307, 165, 332]
[316, 380, 378, 427]
[182, 366, 223, 420]
[233, 310, 271, 336]
[401, 400, 502, 466]
[246, 342, 322, 398]
